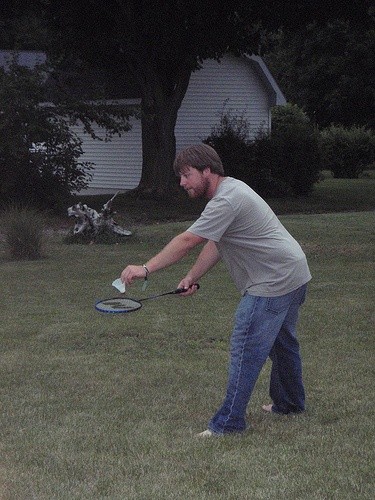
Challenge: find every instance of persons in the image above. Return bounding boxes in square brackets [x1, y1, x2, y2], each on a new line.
[120, 142, 313, 440]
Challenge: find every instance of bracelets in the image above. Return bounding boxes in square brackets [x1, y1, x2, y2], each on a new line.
[142, 264, 150, 281]
